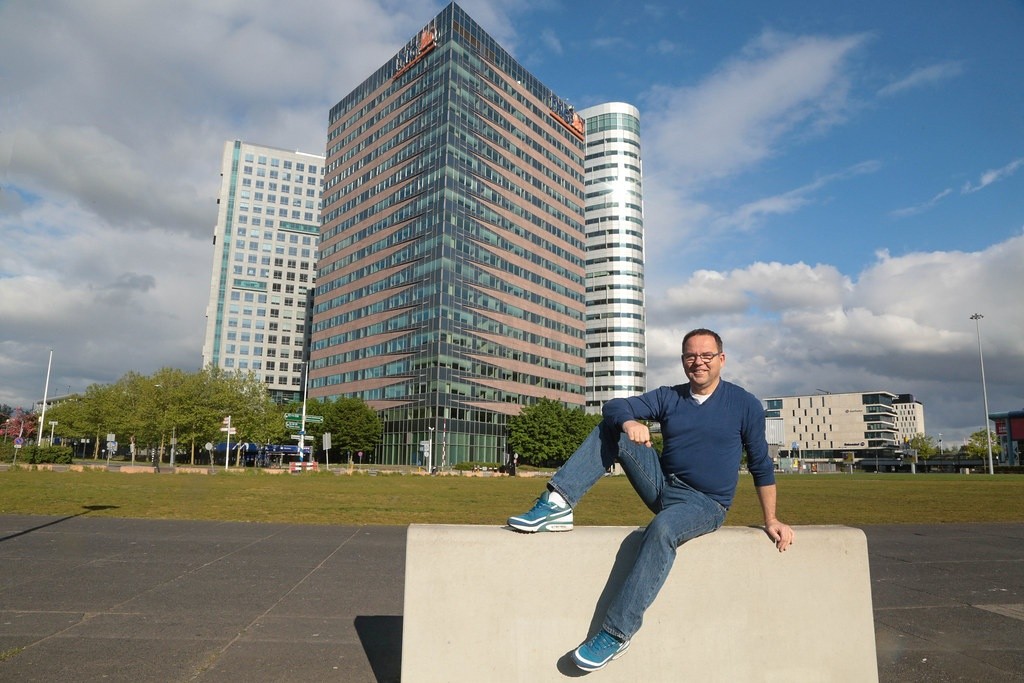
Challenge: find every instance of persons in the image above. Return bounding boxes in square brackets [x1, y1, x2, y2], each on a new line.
[507, 329, 793, 672]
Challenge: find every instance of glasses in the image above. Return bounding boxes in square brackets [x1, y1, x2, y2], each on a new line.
[683, 351, 722, 363]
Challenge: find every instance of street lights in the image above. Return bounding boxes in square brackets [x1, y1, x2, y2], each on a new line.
[969, 313, 994, 476]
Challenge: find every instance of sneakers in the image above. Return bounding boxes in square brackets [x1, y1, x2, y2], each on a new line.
[507, 490, 575, 533]
[570, 628, 630, 672]
